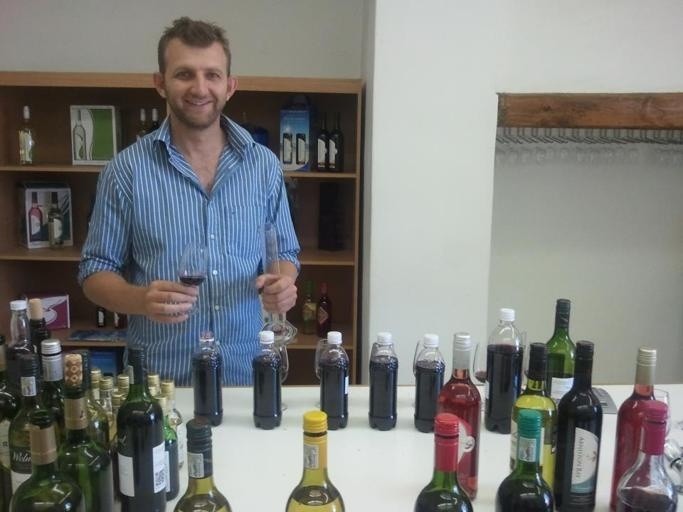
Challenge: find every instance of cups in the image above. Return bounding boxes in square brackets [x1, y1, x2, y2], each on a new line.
[472, 342, 488, 381]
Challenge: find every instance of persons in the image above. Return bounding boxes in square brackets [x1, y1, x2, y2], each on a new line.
[77, 17, 300, 386]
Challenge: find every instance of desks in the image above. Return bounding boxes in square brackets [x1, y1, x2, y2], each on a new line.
[3, 379, 682, 512]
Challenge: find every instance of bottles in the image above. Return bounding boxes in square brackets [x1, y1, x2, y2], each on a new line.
[439, 333, 482, 499]
[240, 111, 252, 134]
[416, 413, 474, 511]
[2, 292, 180, 512]
[86, 197, 95, 231]
[315, 331, 348, 430]
[253, 331, 289, 428]
[545, 298, 576, 405]
[497, 409, 556, 510]
[510, 340, 555, 491]
[326, 110, 344, 172]
[286, 411, 345, 512]
[486, 307, 523, 433]
[315, 112, 330, 171]
[367, 335, 398, 429]
[134, 105, 160, 142]
[73, 108, 88, 161]
[175, 418, 231, 512]
[46, 191, 65, 250]
[607, 348, 671, 511]
[612, 400, 678, 510]
[318, 185, 345, 249]
[16, 104, 36, 167]
[28, 191, 43, 246]
[194, 331, 224, 426]
[557, 340, 602, 512]
[412, 333, 446, 431]
[281, 132, 306, 165]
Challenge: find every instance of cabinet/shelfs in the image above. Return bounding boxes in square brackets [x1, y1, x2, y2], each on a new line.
[1, 70, 362, 387]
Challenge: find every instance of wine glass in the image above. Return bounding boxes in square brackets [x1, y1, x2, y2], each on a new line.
[177, 241, 208, 316]
